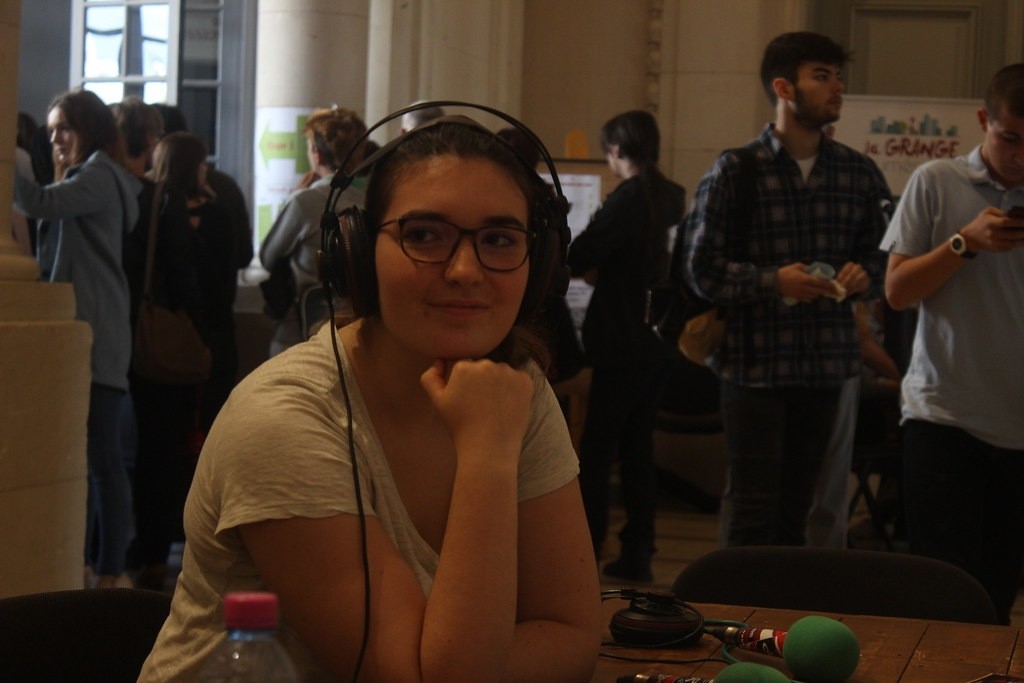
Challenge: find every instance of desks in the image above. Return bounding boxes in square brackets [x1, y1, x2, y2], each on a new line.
[590, 597, 1024, 683]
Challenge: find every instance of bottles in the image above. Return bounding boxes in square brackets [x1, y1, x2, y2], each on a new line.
[197, 592, 301, 683]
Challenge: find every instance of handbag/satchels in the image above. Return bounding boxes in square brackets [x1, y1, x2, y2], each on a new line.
[134, 307, 212, 382]
[679, 307, 725, 365]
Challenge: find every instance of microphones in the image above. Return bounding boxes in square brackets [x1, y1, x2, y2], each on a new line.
[710, 616, 861, 683]
[619, 661, 788, 683]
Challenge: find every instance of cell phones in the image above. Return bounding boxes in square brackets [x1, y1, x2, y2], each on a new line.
[1002, 205, 1024, 230]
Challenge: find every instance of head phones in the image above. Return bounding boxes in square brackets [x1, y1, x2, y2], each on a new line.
[601, 588, 703, 647]
[323, 100, 570, 320]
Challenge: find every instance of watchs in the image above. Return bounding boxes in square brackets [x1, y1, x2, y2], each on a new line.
[948, 230, 978, 260]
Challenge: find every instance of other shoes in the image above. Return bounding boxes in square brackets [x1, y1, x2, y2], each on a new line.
[603, 560, 650, 584]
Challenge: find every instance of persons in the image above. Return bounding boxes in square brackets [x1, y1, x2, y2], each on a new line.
[14, 90, 449, 590]
[568, 110, 688, 584]
[878, 62, 1024, 626]
[678, 30, 901, 547]
[136, 117, 603, 683]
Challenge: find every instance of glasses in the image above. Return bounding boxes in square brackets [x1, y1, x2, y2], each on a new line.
[378, 214, 541, 272]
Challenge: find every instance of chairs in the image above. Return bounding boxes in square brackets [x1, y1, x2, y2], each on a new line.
[0, 589, 173, 683]
[671, 547, 999, 626]
